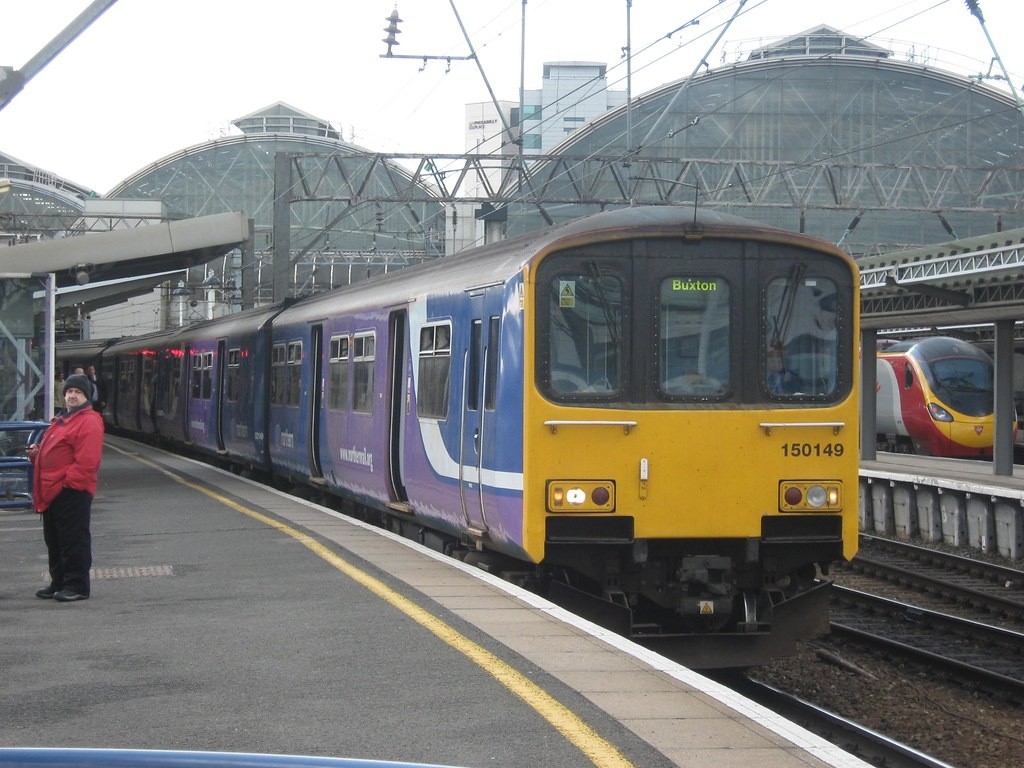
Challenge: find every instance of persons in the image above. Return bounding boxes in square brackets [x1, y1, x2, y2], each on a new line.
[31, 365, 106, 427]
[25, 374, 106, 601]
[766, 343, 804, 395]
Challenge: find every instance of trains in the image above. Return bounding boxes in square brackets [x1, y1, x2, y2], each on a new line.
[869, 331, 1022, 462]
[27, 199, 873, 680]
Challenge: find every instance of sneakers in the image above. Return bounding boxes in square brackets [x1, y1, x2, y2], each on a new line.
[35, 585, 64, 599]
[52, 586, 90, 601]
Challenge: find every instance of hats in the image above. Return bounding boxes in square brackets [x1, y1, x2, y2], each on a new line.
[61, 373, 92, 399]
